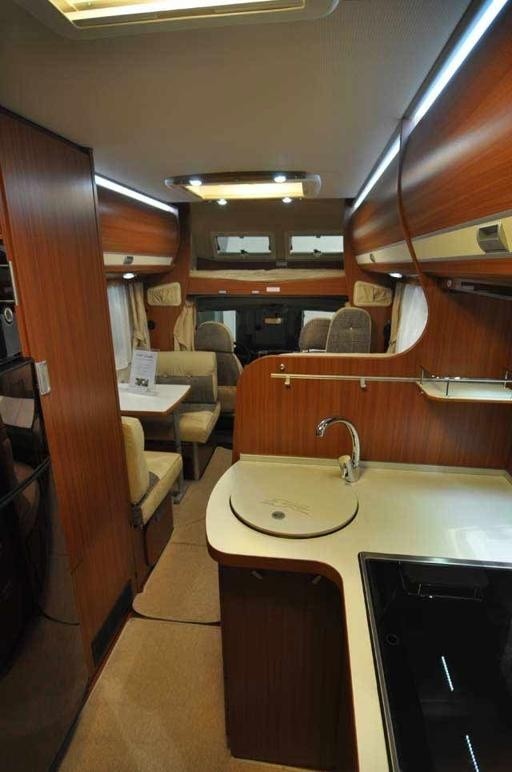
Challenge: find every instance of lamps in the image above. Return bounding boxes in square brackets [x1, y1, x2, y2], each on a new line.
[162, 170, 322, 206]
[12, 0, 341, 41]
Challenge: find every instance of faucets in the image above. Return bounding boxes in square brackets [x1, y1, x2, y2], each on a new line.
[316, 415, 362, 482]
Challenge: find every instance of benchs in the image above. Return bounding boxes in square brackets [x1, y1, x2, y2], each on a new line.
[131, 350, 222, 480]
[120, 416, 183, 594]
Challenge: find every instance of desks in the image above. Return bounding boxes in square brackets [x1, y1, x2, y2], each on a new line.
[116, 383, 193, 504]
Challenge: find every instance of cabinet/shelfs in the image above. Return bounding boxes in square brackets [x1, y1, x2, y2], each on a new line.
[93, 173, 182, 282]
[348, 119, 421, 276]
[399, 1, 512, 284]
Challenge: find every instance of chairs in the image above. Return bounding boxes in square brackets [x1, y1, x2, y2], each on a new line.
[299, 317, 331, 353]
[194, 320, 244, 414]
